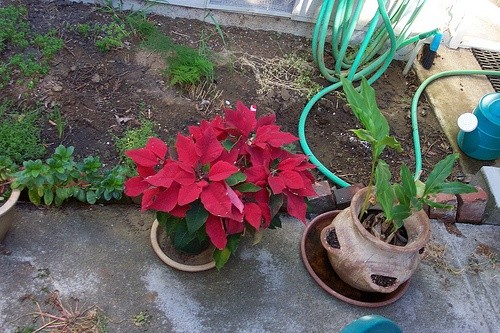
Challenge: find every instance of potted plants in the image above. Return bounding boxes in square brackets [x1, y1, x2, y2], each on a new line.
[317, 74, 471, 297]
[0, 146, 127, 242]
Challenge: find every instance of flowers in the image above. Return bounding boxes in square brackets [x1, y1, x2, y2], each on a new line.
[123, 101, 301, 263]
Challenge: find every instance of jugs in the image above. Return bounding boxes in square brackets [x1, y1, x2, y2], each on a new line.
[457, 92, 500, 160]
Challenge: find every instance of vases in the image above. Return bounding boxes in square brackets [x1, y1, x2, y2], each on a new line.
[146, 214, 229, 272]
